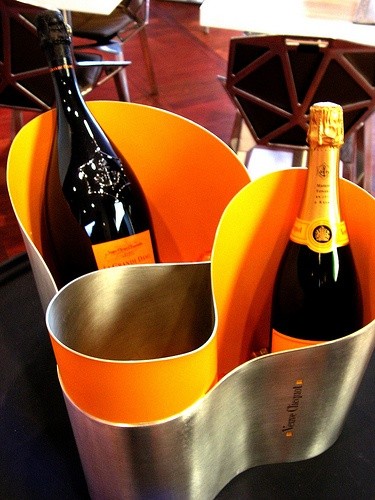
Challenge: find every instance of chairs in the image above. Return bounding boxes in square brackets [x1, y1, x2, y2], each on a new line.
[209, 29, 375, 192]
[0, 0, 165, 152]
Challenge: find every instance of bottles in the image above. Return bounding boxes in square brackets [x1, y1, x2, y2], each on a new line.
[37, 6, 159, 290]
[268, 102, 364, 356]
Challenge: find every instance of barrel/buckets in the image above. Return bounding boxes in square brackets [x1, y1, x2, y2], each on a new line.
[5, 99, 374, 499]
